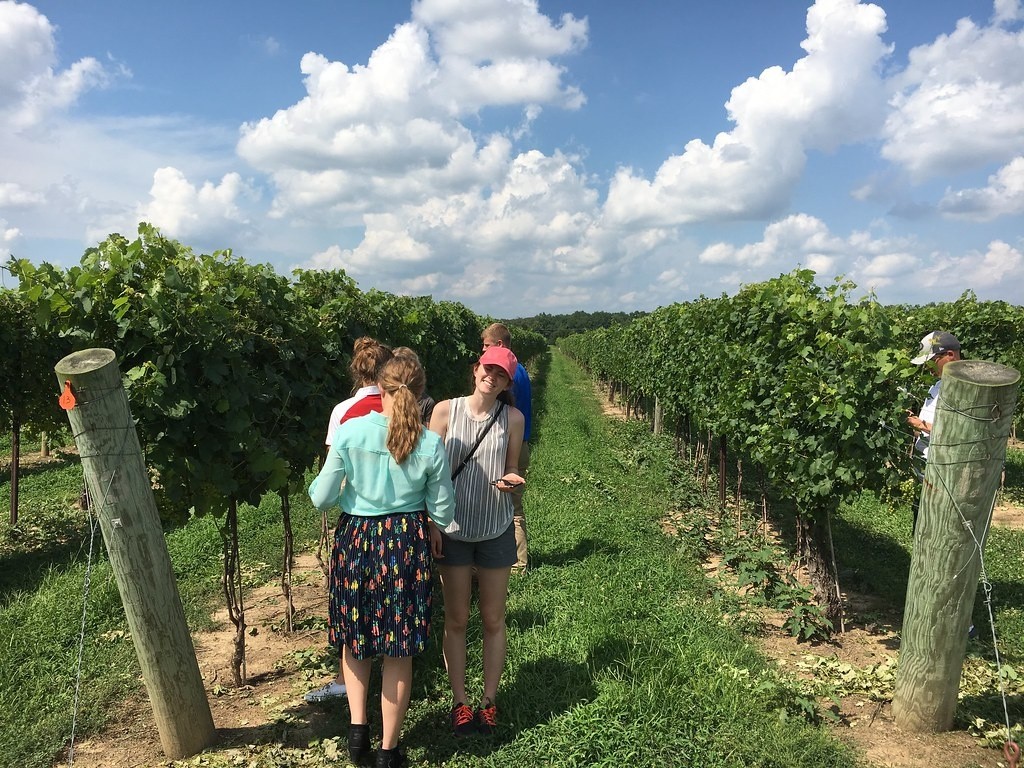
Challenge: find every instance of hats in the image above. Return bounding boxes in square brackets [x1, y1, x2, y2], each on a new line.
[910, 331, 960, 364]
[479, 347, 517, 381]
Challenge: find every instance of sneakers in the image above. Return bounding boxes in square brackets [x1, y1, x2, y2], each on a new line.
[305, 680, 348, 702]
[476, 703, 497, 736]
[451, 702, 475, 737]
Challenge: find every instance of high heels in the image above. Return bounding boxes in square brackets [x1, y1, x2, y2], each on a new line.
[376, 743, 404, 768]
[348, 724, 371, 762]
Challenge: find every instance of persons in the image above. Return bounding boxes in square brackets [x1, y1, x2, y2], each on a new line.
[482, 323, 538, 576]
[306, 331, 459, 768]
[427, 345, 527, 735]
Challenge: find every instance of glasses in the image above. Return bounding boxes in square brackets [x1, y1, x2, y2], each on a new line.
[921, 353, 944, 372]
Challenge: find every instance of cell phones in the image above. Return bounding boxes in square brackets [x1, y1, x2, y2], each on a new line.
[489, 479, 523, 487]
